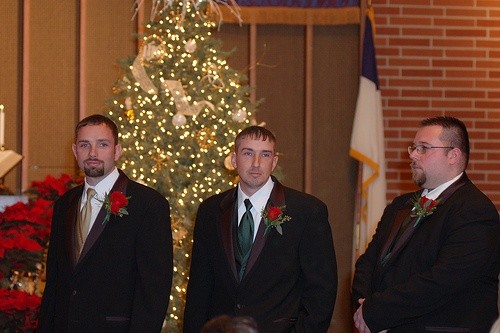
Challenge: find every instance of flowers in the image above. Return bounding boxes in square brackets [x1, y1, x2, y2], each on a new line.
[90, 190, 134, 226]
[408, 191, 444, 227]
[260, 202, 292, 236]
[0, 172, 84, 333]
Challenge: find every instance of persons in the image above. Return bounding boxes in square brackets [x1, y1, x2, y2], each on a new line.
[181, 125, 339, 332]
[352, 116, 500, 333]
[37, 114, 174, 332]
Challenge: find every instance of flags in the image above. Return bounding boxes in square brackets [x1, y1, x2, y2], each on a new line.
[350, 11, 387, 260]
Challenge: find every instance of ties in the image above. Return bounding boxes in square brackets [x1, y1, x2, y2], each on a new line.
[74, 189, 96, 262]
[232, 200, 255, 283]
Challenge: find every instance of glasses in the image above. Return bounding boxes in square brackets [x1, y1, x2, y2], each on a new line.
[408, 144, 456, 155]
